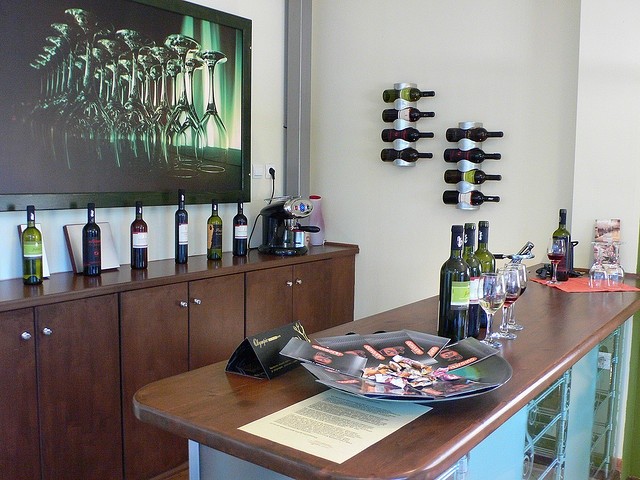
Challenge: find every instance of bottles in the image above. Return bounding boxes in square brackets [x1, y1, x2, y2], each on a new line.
[175, 189, 188, 265]
[382, 109, 436, 124]
[458, 223, 482, 339]
[495, 241, 534, 275]
[130, 201, 148, 271]
[381, 147, 434, 162]
[309, 194, 326, 246]
[437, 225, 470, 345]
[381, 128, 435, 142]
[232, 198, 248, 257]
[446, 128, 504, 142]
[474, 220, 495, 330]
[443, 168, 502, 185]
[552, 208, 573, 279]
[206, 200, 223, 260]
[383, 89, 436, 104]
[443, 147, 502, 163]
[82, 202, 102, 277]
[21, 204, 43, 286]
[443, 189, 501, 206]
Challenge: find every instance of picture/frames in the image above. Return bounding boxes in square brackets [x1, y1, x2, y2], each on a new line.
[0, 0, 250, 213]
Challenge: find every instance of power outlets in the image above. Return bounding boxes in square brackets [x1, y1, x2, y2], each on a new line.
[266, 164, 276, 178]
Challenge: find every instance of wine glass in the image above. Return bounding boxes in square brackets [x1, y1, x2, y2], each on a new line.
[588, 243, 609, 285]
[498, 263, 527, 331]
[21, 8, 229, 178]
[546, 238, 566, 285]
[477, 273, 505, 348]
[488, 269, 520, 340]
[609, 241, 625, 284]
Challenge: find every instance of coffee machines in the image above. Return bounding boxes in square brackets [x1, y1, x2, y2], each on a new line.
[257, 197, 320, 256]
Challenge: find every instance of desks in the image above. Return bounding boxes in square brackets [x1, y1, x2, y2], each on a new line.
[131, 262, 640, 480]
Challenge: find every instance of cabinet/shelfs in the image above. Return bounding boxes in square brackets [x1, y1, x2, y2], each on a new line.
[246, 258, 329, 336]
[0, 293, 124, 479]
[119, 272, 246, 480]
